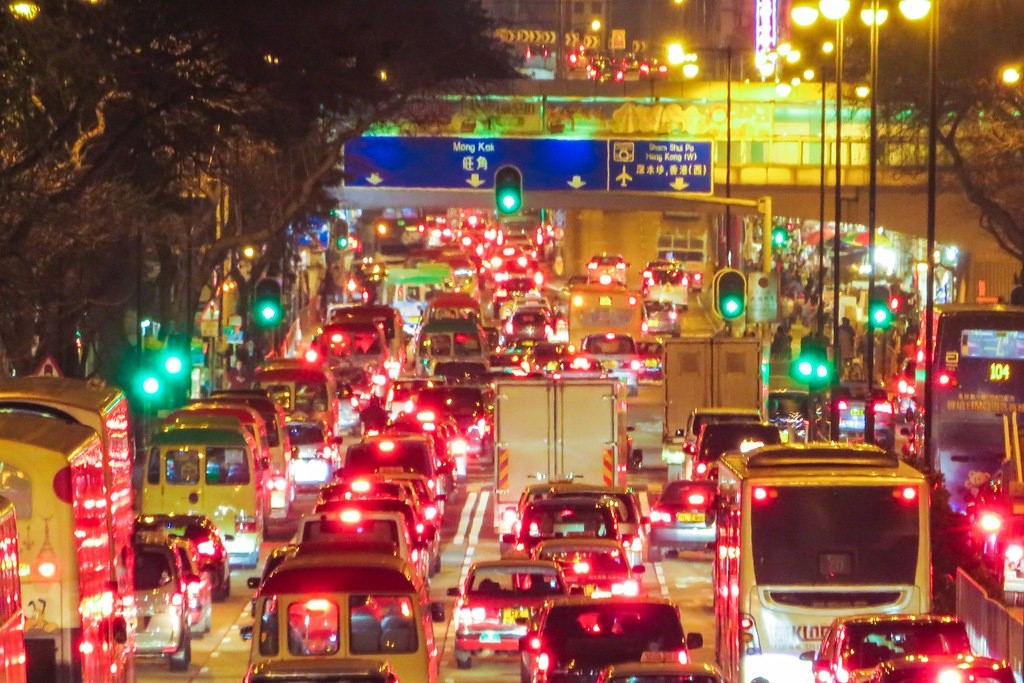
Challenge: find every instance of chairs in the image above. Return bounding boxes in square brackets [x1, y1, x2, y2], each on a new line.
[227, 472, 247, 483]
[229, 462, 247, 472]
[350, 613, 377, 629]
[207, 464, 226, 472]
[382, 627, 411, 654]
[264, 623, 304, 654]
[206, 472, 225, 483]
[351, 629, 380, 651]
[381, 613, 414, 631]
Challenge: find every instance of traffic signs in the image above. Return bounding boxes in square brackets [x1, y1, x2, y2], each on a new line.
[340, 132, 609, 193]
[607, 137, 714, 196]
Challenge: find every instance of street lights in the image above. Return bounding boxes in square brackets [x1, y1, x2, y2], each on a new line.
[789, 0, 934, 473]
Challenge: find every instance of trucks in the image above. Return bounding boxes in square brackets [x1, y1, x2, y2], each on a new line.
[494, 381, 635, 557]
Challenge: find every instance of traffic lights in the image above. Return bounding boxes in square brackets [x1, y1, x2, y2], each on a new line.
[253, 278, 282, 328]
[716, 270, 746, 320]
[136, 350, 186, 399]
[493, 164, 525, 216]
[798, 336, 829, 380]
[869, 285, 891, 327]
[335, 218, 349, 250]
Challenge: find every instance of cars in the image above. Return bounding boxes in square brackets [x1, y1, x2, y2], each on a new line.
[130, 208, 1023, 683]
[525, 41, 668, 83]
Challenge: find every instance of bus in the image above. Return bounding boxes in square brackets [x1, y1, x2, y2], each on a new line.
[0, 374, 139, 683]
[704, 442, 931, 683]
[910, 301, 1023, 516]
